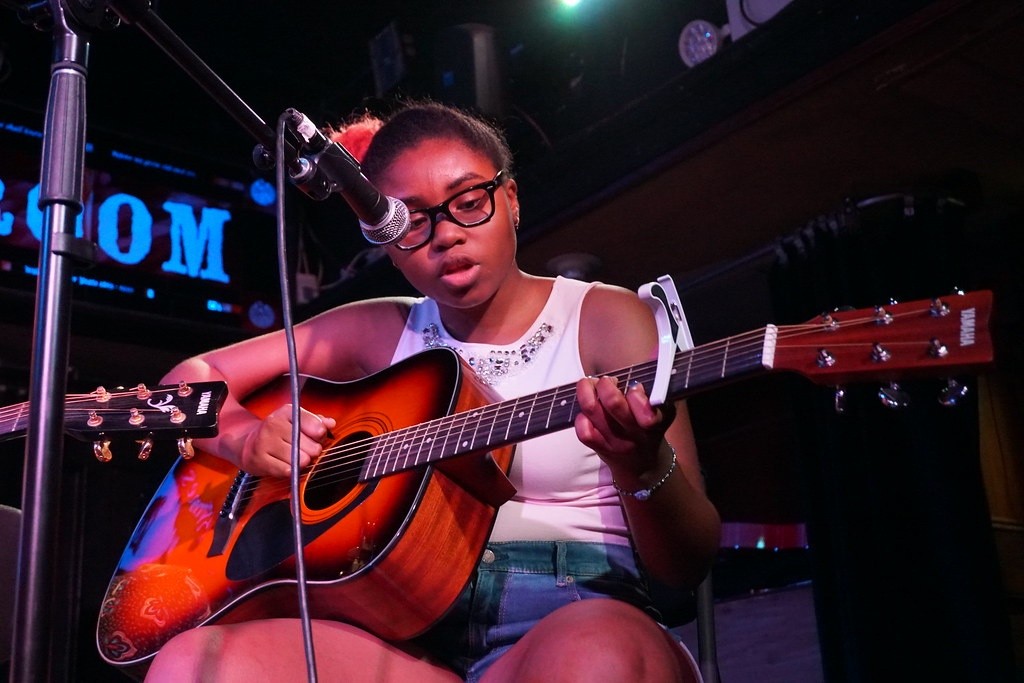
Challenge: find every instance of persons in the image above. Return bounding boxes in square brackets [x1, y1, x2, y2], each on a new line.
[143, 104, 723, 683]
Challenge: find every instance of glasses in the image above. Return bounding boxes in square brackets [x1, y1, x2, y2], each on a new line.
[393, 168, 505, 250]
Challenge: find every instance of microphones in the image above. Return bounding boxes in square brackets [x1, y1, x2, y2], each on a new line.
[286, 108, 411, 246]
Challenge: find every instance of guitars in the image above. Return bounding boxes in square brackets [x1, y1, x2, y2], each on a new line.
[92, 284, 1000, 668]
[0, 379, 231, 464]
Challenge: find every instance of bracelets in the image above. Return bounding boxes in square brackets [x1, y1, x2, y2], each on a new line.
[612, 441, 676, 499]
